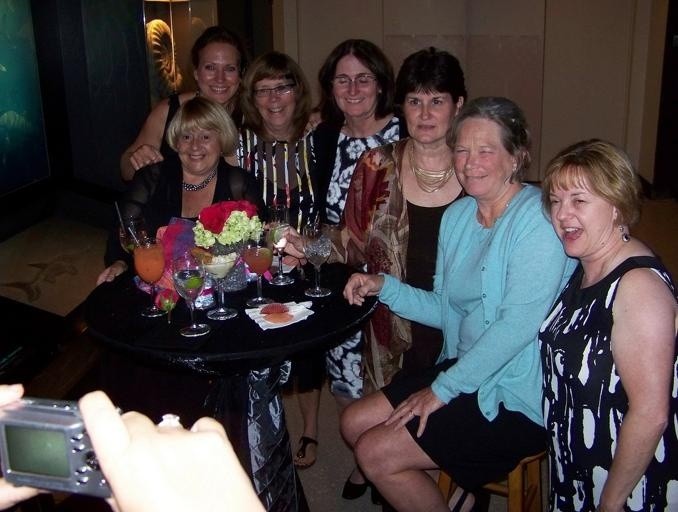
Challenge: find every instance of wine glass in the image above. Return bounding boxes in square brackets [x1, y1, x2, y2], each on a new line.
[244, 234, 275, 305]
[198, 253, 242, 319]
[135, 237, 168, 319]
[269, 222, 293, 286]
[301, 222, 334, 297]
[170, 256, 212, 337]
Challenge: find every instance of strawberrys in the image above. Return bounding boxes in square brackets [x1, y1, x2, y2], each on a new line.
[155, 290, 180, 311]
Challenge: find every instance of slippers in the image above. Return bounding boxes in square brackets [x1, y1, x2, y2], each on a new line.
[293, 437, 318, 469]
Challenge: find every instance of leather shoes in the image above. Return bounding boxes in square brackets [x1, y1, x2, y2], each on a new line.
[342, 470, 368, 500]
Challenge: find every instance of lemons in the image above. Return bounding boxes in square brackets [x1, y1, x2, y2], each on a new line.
[187, 276, 203, 289]
[270, 226, 283, 244]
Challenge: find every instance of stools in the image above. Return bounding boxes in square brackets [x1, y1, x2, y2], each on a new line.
[439, 451, 549, 512]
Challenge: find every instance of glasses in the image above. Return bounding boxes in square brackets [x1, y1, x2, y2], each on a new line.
[256, 84, 295, 97]
[332, 74, 377, 86]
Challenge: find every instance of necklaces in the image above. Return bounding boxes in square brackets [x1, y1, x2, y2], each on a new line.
[182, 167, 215, 191]
[407, 141, 455, 193]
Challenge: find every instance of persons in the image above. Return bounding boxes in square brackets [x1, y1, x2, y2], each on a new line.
[305, 36, 407, 506]
[223, 54, 321, 469]
[536, 136, 677, 512]
[336, 95, 580, 512]
[95, 93, 269, 288]
[0, 377, 276, 512]
[271, 47, 468, 396]
[118, 23, 245, 182]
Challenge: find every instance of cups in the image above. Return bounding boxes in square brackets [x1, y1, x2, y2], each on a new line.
[119, 215, 152, 253]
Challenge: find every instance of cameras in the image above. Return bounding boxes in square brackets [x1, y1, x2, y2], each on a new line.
[0, 398, 115, 498]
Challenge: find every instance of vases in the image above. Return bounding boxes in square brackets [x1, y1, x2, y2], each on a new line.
[212, 240, 248, 292]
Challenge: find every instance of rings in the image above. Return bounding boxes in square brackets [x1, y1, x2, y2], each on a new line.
[158, 410, 184, 432]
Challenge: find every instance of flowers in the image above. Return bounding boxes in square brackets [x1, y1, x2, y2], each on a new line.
[191, 200, 266, 250]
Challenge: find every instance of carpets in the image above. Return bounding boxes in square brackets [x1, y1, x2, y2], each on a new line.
[0, 219, 112, 317]
